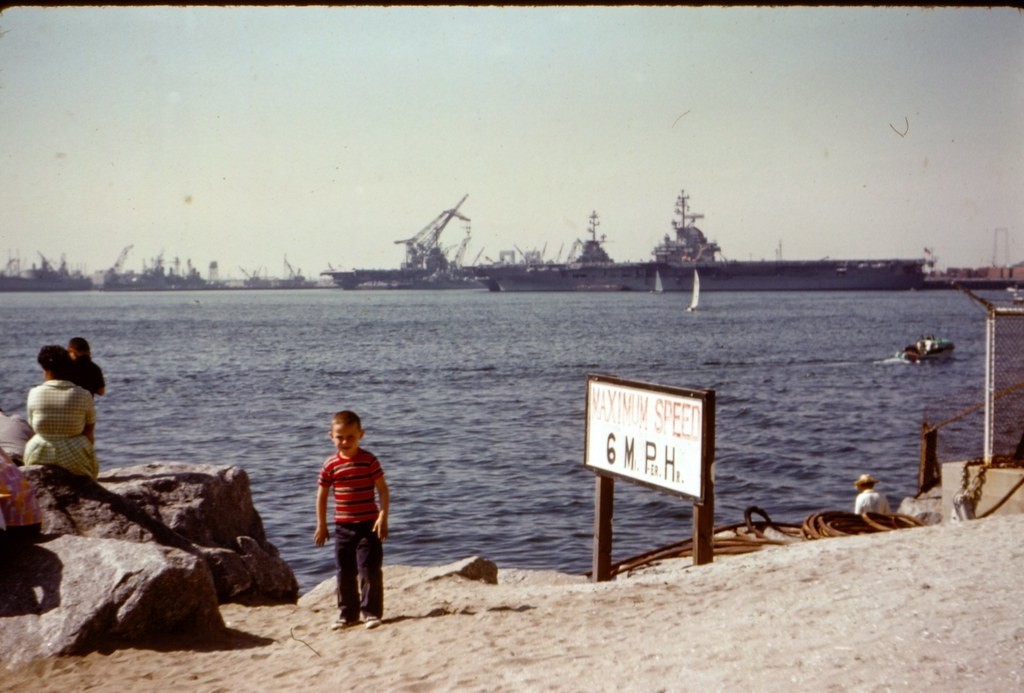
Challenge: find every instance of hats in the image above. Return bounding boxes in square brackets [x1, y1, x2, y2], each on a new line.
[855, 474, 880, 489]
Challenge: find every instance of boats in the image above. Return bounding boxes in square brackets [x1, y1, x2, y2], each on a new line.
[317, 188, 479, 290]
[894, 330, 956, 364]
[468, 190, 932, 297]
[0, 253, 315, 296]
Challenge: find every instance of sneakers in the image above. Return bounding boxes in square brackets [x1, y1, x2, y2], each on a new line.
[365, 616, 380, 628]
[331, 615, 359, 629]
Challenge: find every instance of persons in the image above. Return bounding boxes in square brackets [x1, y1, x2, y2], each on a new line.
[313, 411, 389, 630]
[854, 474, 890, 516]
[23, 339, 105, 480]
